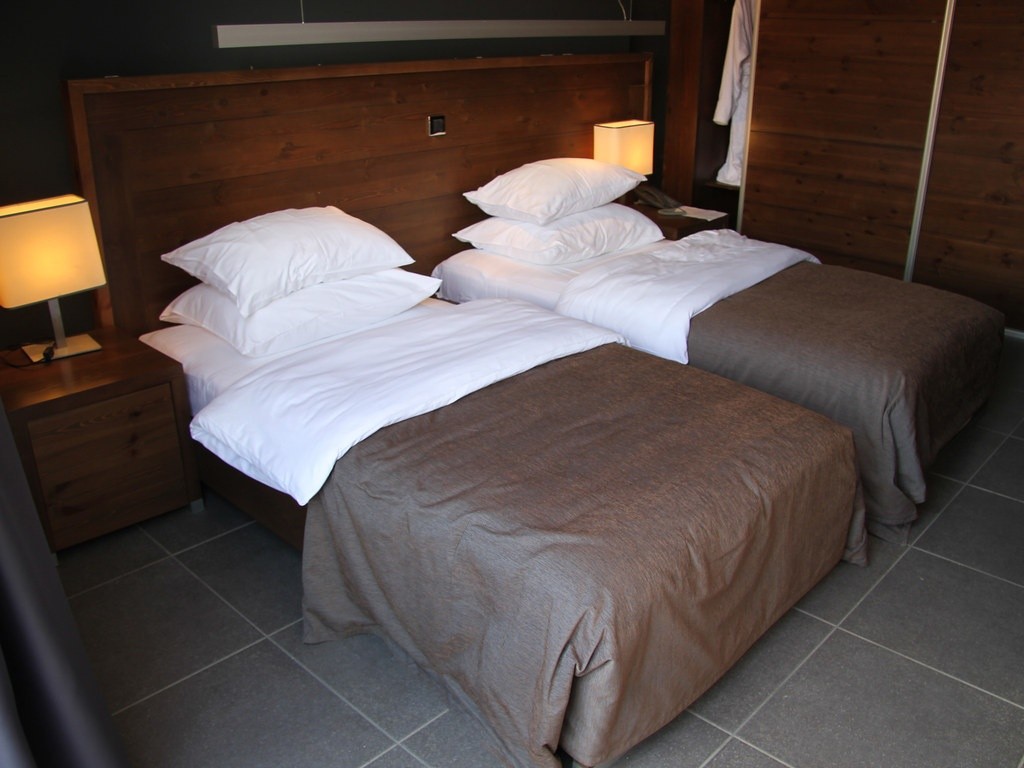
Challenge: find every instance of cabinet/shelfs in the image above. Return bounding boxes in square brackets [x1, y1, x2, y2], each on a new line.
[662, 0, 1024, 320]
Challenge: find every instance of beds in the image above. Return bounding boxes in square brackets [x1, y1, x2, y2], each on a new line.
[138, 281, 858, 767]
[432, 215, 1007, 532]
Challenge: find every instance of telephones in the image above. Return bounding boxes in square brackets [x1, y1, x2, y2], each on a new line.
[635, 185, 681, 210]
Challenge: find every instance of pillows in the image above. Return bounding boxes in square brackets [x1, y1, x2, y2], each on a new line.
[161, 267, 443, 358]
[160, 204, 416, 319]
[461, 157, 649, 228]
[452, 202, 667, 265]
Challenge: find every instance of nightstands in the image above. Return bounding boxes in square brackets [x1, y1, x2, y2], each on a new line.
[0, 325, 202, 554]
[642, 206, 731, 241]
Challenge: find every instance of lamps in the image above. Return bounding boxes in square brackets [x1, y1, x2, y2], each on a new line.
[592, 119, 654, 176]
[1, 193, 108, 364]
